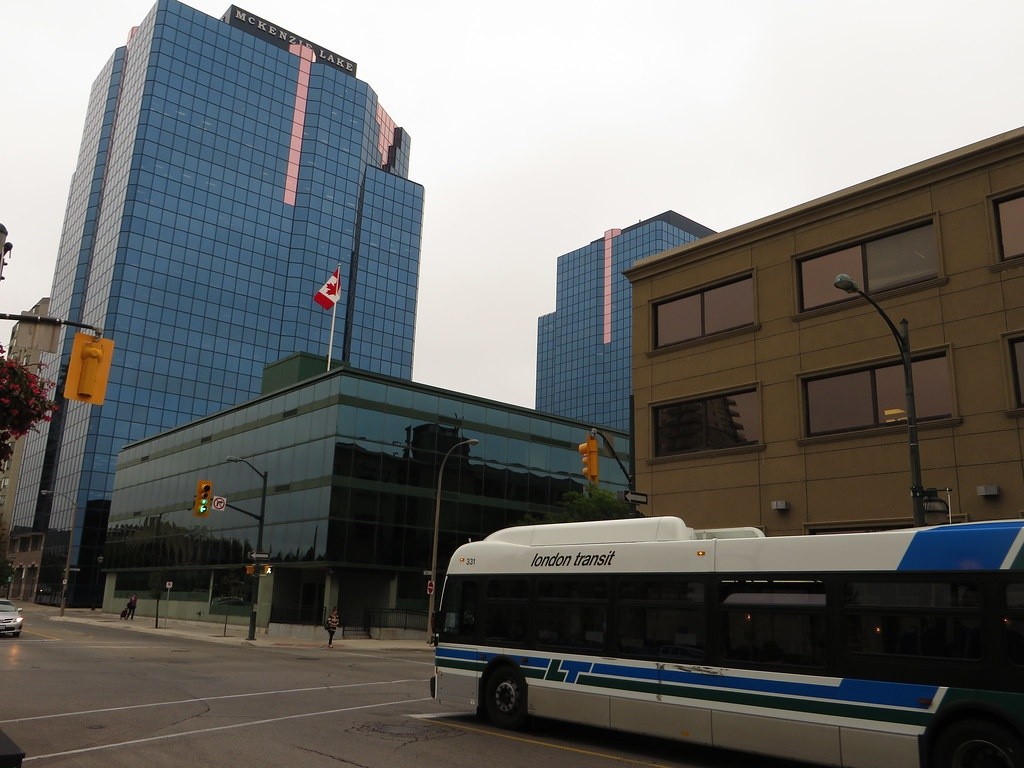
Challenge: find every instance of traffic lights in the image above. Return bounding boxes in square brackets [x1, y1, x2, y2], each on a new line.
[197, 483, 211, 515]
[264, 564, 274, 578]
[241, 565, 255, 577]
[579, 443, 588, 478]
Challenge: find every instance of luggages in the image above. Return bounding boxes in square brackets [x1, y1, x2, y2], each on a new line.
[120, 608, 128, 619]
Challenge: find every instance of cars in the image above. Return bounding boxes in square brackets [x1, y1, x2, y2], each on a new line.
[0, 598, 24, 636]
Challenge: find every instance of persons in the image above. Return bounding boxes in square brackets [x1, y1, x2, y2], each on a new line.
[126, 593, 137, 621]
[325, 609, 340, 648]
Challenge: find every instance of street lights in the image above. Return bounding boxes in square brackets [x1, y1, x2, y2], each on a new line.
[193, 456, 274, 640]
[6, 554, 16, 599]
[427, 439, 480, 643]
[834, 272, 953, 528]
[40, 490, 77, 616]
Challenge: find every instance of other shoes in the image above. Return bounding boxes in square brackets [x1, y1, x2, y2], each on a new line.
[328, 645, 333, 648]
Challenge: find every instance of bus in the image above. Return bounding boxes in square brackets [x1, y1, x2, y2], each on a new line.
[429, 516, 1024, 768]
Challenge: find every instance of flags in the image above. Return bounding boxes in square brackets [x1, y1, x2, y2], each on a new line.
[313, 269, 341, 311]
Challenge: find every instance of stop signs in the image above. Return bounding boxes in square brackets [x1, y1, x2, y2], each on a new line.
[427, 581, 434, 595]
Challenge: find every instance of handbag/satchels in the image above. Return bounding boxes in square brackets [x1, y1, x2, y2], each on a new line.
[325, 619, 330, 630]
[127, 603, 132, 609]
